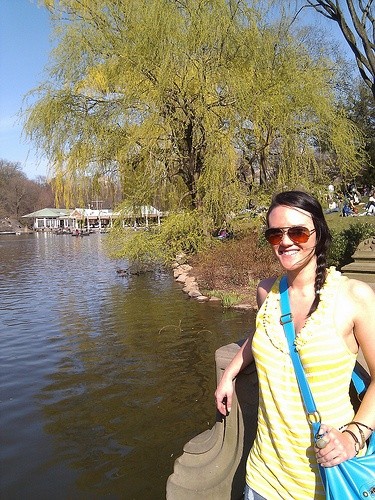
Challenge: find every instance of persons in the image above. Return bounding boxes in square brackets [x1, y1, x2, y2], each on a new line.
[213, 184, 375, 245]
[214, 192, 374, 500]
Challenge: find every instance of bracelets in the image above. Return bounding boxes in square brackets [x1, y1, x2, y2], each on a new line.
[339, 422, 374, 455]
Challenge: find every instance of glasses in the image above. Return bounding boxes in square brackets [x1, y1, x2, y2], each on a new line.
[264, 227, 315, 245]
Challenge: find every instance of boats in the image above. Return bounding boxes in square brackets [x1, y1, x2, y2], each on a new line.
[54, 227, 111, 238]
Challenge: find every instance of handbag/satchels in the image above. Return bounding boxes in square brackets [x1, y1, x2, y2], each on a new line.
[310, 423, 375, 500]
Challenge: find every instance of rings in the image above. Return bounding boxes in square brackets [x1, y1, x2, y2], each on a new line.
[315, 438, 327, 449]
